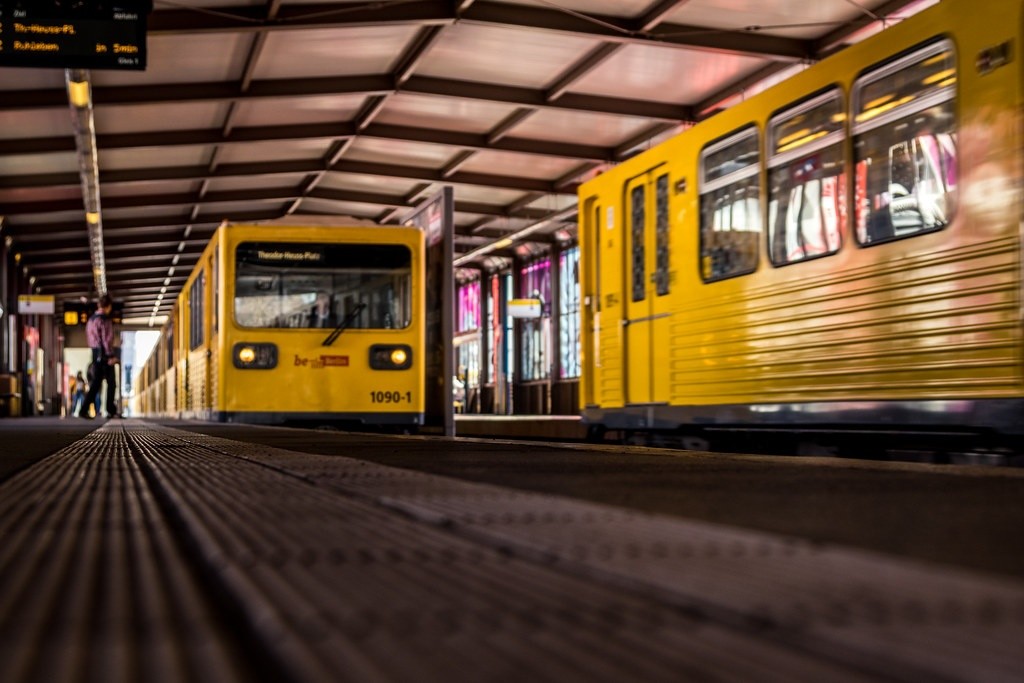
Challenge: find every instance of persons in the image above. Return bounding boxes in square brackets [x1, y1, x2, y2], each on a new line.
[867, 161, 947, 241]
[69, 361, 103, 418]
[79, 297, 126, 419]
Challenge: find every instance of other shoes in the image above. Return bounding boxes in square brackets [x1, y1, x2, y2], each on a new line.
[106, 412, 126, 419]
[79, 411, 95, 420]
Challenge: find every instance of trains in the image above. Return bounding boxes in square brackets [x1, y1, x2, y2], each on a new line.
[576, 2, 1024, 460]
[135, 218, 430, 434]
